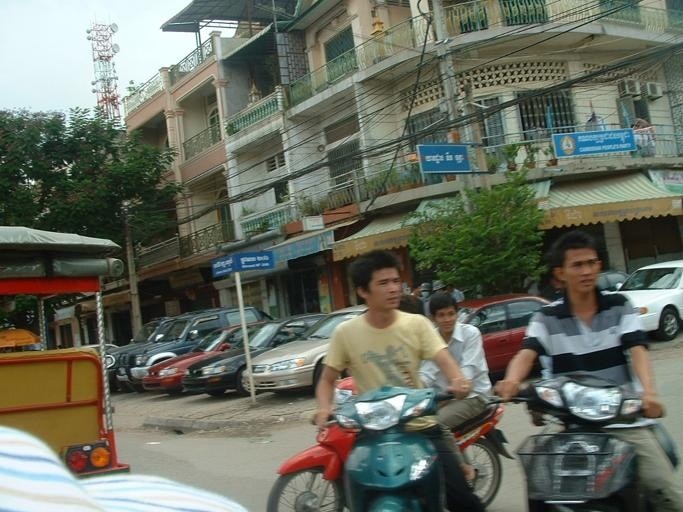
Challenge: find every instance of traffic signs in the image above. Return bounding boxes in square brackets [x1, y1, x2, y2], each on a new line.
[211, 254, 235, 279]
[415, 143, 473, 173]
[235, 250, 275, 272]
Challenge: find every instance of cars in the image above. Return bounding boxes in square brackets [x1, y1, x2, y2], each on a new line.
[608, 259, 683, 342]
[143, 320, 264, 395]
[81, 344, 119, 384]
[181, 311, 331, 397]
[246, 303, 371, 394]
[594, 270, 642, 290]
[333, 294, 558, 410]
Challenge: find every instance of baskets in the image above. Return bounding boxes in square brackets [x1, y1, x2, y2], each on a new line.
[515, 433, 639, 501]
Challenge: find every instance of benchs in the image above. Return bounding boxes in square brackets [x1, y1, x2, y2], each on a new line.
[0, 348, 105, 456]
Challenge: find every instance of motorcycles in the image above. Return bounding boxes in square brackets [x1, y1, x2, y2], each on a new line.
[263, 376, 515, 512]
[312, 383, 467, 512]
[484, 373, 667, 512]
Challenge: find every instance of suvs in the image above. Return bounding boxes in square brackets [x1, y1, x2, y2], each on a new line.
[102, 315, 166, 395]
[116, 306, 262, 394]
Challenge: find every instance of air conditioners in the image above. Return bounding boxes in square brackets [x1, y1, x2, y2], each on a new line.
[641, 82, 663, 100]
[617, 79, 641, 98]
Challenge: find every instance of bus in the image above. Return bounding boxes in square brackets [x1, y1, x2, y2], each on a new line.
[0, 225, 132, 478]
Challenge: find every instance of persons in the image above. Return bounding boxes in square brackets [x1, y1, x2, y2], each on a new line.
[424, 291, 494, 492]
[410, 281, 465, 304]
[491, 233, 683, 510]
[317, 251, 486, 512]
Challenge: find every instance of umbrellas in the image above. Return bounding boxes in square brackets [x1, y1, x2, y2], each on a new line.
[0, 325, 43, 352]
